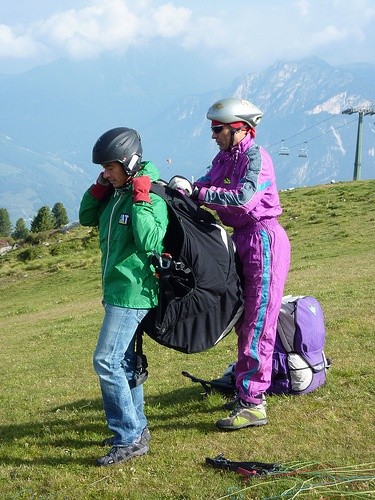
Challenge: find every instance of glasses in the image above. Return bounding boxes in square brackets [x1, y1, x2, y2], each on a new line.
[211, 125, 224, 133]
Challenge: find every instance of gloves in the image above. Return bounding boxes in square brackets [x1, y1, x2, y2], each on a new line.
[152, 178, 168, 187]
[168, 176, 200, 201]
[90, 171, 110, 201]
[132, 176, 152, 204]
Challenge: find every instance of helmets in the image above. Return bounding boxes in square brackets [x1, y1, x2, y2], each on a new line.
[207, 97, 264, 132]
[92, 127, 142, 176]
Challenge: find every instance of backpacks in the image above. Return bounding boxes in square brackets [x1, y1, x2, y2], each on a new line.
[266, 295, 330, 395]
[127, 182, 247, 355]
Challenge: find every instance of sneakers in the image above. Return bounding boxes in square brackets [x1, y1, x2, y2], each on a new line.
[96, 434, 150, 466]
[222, 394, 268, 414]
[102, 428, 151, 446]
[216, 400, 268, 429]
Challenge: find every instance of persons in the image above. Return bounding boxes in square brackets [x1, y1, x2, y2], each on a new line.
[154, 98, 291, 430]
[79, 128, 169, 466]
